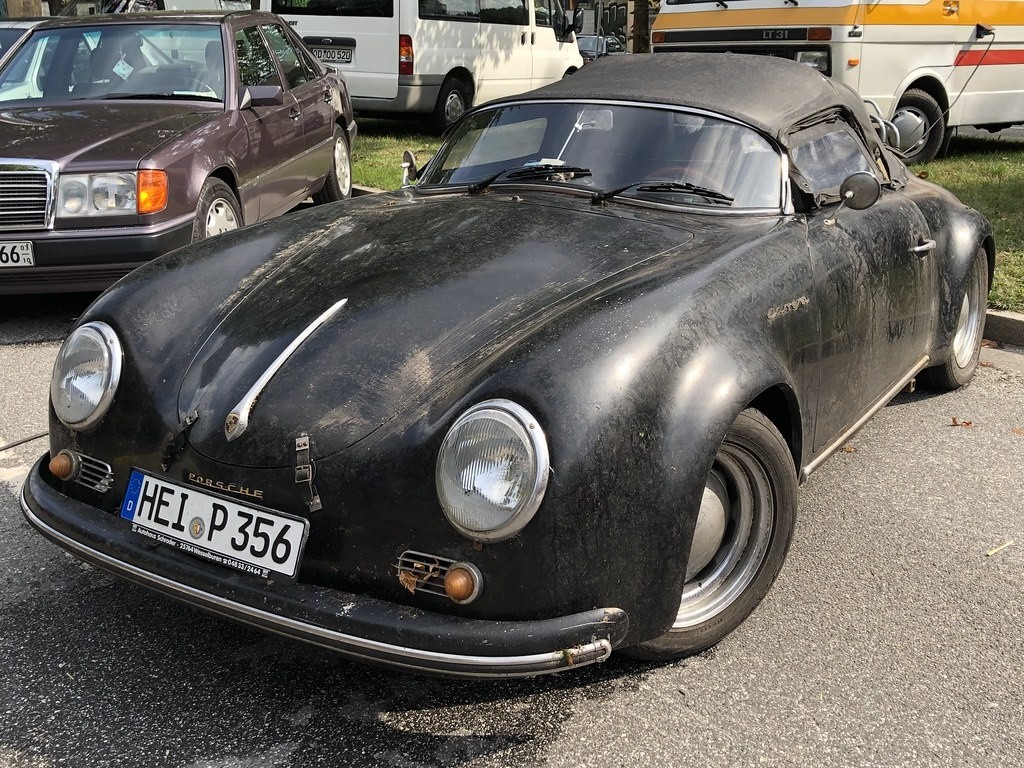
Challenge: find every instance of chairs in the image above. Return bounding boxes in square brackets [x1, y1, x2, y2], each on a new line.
[71, 47, 125, 97]
[205, 39, 251, 88]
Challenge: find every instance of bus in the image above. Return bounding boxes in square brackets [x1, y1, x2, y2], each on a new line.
[649, 0, 1024, 167]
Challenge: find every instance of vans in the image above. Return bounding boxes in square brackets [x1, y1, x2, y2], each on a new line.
[259, 0, 585, 141]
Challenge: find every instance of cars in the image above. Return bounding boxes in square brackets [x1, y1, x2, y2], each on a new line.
[0, 0, 253, 102]
[18, 50, 996, 677]
[0, 10, 360, 297]
